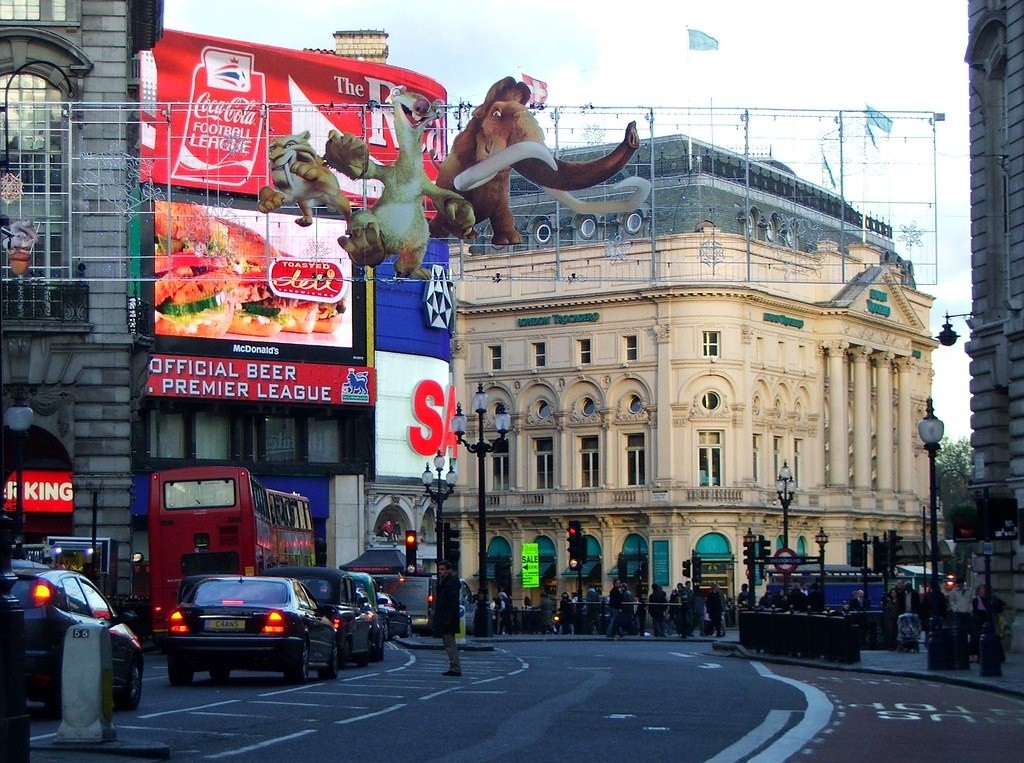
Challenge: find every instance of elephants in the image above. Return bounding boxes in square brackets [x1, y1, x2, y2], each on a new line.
[428, 76, 652, 246]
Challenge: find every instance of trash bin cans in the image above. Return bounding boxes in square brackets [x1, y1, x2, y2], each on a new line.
[941, 625, 971, 669]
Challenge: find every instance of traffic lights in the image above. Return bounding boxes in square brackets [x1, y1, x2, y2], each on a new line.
[743, 535, 771, 565]
[405, 530, 416, 576]
[692, 557, 702, 582]
[567, 521, 582, 571]
[682, 560, 691, 578]
[889, 530, 903, 563]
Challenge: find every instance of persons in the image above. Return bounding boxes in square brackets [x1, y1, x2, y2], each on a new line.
[431, 561, 462, 676]
[491, 578, 989, 653]
[383, 520, 401, 541]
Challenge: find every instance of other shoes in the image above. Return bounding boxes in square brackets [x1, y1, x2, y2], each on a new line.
[620, 633, 626, 638]
[442, 671, 461, 676]
[607, 635, 615, 637]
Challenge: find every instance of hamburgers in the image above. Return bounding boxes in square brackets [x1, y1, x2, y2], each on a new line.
[154, 201, 346, 339]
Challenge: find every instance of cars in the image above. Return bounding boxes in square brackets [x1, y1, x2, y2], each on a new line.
[375, 591, 413, 641]
[429, 575, 479, 633]
[259, 566, 381, 669]
[161, 574, 339, 687]
[176, 574, 243, 605]
[347, 572, 380, 616]
[11, 567, 144, 711]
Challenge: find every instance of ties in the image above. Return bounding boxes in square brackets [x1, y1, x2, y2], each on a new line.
[860, 599, 863, 607]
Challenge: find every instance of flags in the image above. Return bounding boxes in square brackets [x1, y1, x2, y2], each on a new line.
[521, 73, 548, 109]
[868, 106, 893, 152]
[688, 29, 718, 51]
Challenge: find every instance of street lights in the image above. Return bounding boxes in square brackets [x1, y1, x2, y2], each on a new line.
[5, 384, 33, 558]
[421, 450, 457, 637]
[776, 461, 797, 597]
[451, 377, 511, 637]
[918, 397, 954, 671]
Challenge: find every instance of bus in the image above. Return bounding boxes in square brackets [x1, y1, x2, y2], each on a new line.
[147, 467, 317, 649]
